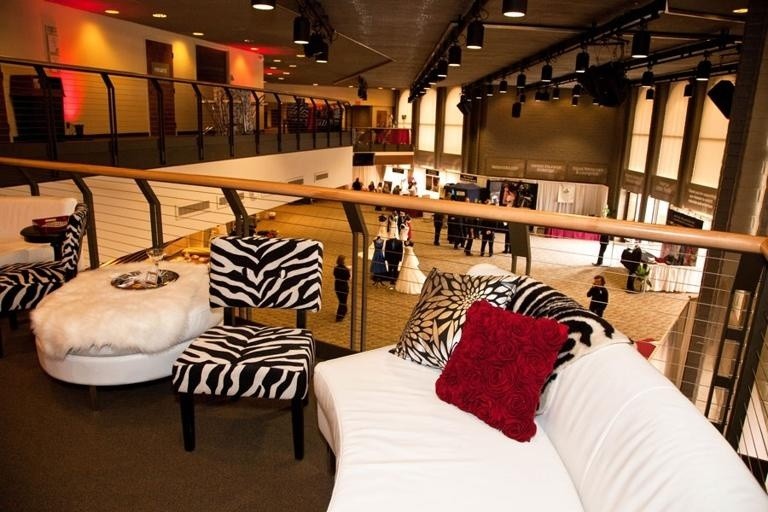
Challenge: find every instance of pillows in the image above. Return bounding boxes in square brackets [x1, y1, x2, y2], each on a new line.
[389, 268, 570, 443]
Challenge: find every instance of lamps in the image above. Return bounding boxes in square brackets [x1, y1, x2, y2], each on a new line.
[293, 0, 335, 64]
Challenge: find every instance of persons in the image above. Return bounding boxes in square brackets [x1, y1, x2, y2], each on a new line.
[395, 237, 430, 295]
[351, 176, 513, 259]
[370, 236, 388, 287]
[593, 234, 610, 266]
[333, 255, 350, 322]
[502, 183, 516, 226]
[625, 244, 643, 293]
[384, 234, 403, 284]
[663, 250, 678, 264]
[587, 275, 609, 318]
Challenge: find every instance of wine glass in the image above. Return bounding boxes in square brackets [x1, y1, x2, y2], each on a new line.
[146, 248, 167, 278]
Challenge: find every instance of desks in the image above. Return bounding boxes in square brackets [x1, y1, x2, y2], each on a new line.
[36, 263, 227, 412]
[20, 225, 86, 261]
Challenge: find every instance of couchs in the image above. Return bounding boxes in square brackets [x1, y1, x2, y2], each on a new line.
[312, 261, 768, 512]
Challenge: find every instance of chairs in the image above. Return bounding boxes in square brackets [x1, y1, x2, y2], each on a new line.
[173, 235, 324, 461]
[0, 203, 88, 361]
